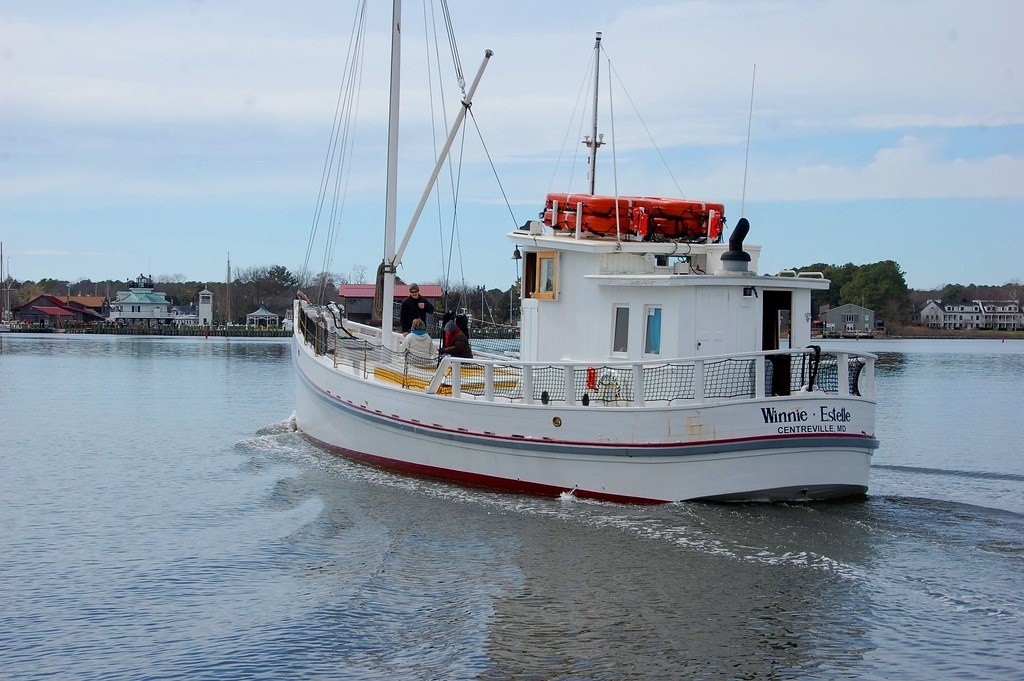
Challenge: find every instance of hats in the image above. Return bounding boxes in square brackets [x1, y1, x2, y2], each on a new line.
[409, 283, 419, 291]
[444, 320, 457, 333]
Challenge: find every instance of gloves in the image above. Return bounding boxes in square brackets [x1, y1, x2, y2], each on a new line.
[438, 347, 445, 355]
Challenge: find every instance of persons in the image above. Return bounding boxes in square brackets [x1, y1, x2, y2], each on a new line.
[437, 321, 473, 365]
[401, 319, 434, 364]
[400, 283, 434, 337]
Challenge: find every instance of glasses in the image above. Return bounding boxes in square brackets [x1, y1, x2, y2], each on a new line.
[410, 290, 419, 293]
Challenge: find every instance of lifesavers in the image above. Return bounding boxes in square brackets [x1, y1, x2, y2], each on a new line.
[539, 190, 726, 238]
[595, 371, 622, 399]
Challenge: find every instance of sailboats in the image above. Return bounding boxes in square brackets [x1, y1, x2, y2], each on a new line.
[0, 242, 17, 331]
[288, 0, 880, 506]
